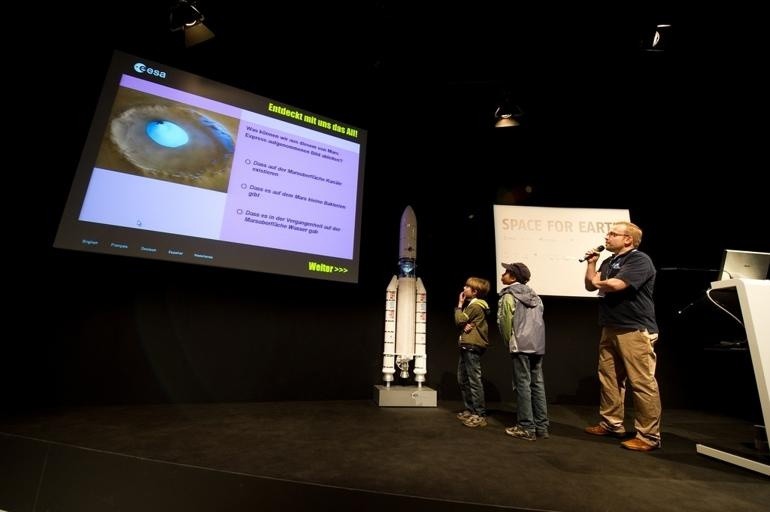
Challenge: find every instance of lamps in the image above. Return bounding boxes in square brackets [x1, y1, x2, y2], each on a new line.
[169, 0, 215, 48]
[494, 101, 524, 128]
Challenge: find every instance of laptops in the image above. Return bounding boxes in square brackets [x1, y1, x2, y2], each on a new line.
[717, 249, 770, 280]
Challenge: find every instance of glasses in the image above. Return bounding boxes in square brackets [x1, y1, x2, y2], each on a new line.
[607, 231, 631, 238]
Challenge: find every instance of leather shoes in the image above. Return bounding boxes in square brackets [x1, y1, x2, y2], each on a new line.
[583, 425, 626, 438]
[621, 438, 658, 451]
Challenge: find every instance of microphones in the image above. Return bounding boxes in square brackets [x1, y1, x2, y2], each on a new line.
[579, 245, 605, 262]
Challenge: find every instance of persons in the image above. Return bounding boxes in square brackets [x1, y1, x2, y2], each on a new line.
[584, 222, 663, 452]
[495, 262, 551, 441]
[453, 277, 492, 428]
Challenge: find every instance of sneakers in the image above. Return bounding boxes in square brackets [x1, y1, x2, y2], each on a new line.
[456, 410, 472, 421]
[461, 414, 489, 428]
[536, 429, 551, 439]
[504, 425, 537, 441]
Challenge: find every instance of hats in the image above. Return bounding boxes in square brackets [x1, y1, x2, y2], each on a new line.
[501, 262, 531, 285]
